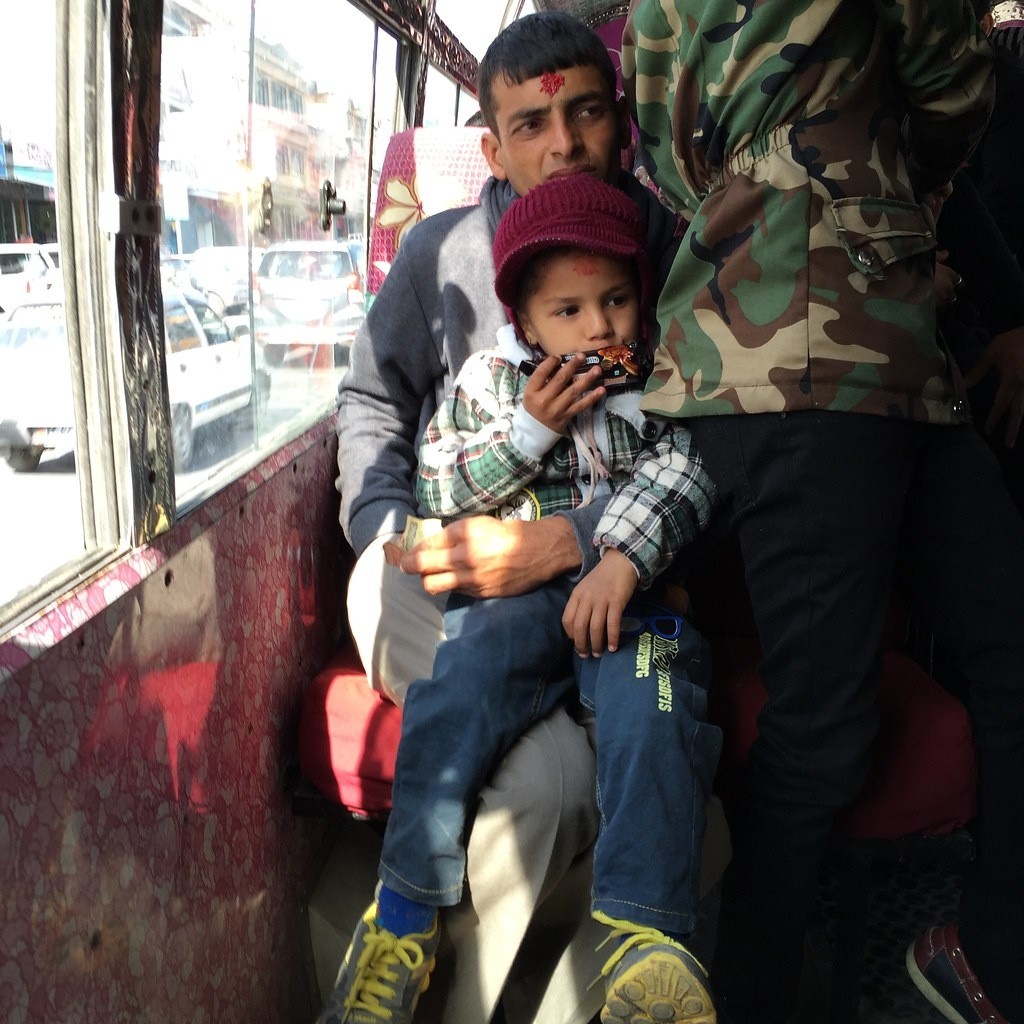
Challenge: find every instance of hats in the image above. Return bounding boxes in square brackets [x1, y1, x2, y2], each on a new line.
[492, 172, 653, 361]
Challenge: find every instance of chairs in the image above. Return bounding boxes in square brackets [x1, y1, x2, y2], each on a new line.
[698, 548, 980, 1021]
[302, 122, 499, 823]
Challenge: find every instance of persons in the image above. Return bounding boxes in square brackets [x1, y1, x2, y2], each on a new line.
[317, 170, 722, 1023]
[534, 0, 1024, 1024]
[332, 11, 736, 1023]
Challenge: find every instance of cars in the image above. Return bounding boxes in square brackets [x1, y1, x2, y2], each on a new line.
[190, 245, 262, 291]
[0, 242, 62, 275]
[159, 250, 196, 280]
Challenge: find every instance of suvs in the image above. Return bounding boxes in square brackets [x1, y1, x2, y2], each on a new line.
[252, 235, 373, 367]
[0, 279, 270, 472]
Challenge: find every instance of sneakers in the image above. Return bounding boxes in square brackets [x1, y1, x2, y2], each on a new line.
[905, 925, 1024, 1024]
[315, 900, 441, 1024]
[587, 911, 720, 1024]
